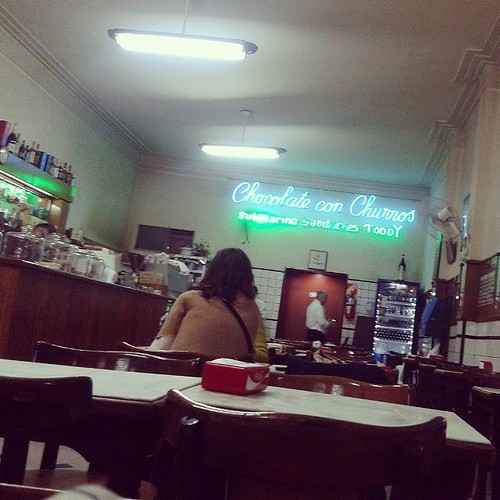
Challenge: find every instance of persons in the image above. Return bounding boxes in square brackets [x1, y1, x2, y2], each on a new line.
[251, 285, 266, 321]
[306, 290, 336, 344]
[32, 223, 57, 239]
[136, 248, 267, 500]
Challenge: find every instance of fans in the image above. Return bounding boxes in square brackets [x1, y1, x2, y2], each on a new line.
[416, 196, 471, 256]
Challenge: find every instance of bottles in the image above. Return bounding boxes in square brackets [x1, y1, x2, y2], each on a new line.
[398, 254, 406, 281]
[6, 122, 41, 167]
[49, 156, 74, 186]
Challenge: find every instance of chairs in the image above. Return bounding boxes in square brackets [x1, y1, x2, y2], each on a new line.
[180, 385, 496, 499]
[0, 337, 500, 499]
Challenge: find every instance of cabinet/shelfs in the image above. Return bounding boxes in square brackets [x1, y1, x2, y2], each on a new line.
[168, 254, 206, 287]
[1, 149, 76, 234]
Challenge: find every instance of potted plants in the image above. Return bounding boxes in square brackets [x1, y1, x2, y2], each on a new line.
[191, 239, 210, 257]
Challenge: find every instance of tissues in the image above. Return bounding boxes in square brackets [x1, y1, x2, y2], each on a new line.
[320, 347, 334, 354]
[375, 361, 391, 372]
[429, 355, 444, 361]
[479, 361, 493, 371]
[201, 359, 271, 395]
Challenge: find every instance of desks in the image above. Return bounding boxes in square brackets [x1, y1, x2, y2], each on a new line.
[435, 368, 464, 383]
[471, 385, 500, 446]
[0, 357, 203, 411]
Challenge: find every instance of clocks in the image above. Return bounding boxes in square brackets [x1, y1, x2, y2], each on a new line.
[308, 249, 328, 270]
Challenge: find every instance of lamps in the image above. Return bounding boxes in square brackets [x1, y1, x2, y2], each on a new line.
[107, 1, 258, 63]
[199, 109, 287, 159]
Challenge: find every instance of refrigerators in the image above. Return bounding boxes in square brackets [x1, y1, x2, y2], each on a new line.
[371, 278, 421, 355]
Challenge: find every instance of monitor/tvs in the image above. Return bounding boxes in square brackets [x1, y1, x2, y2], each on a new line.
[135, 224, 195, 254]
